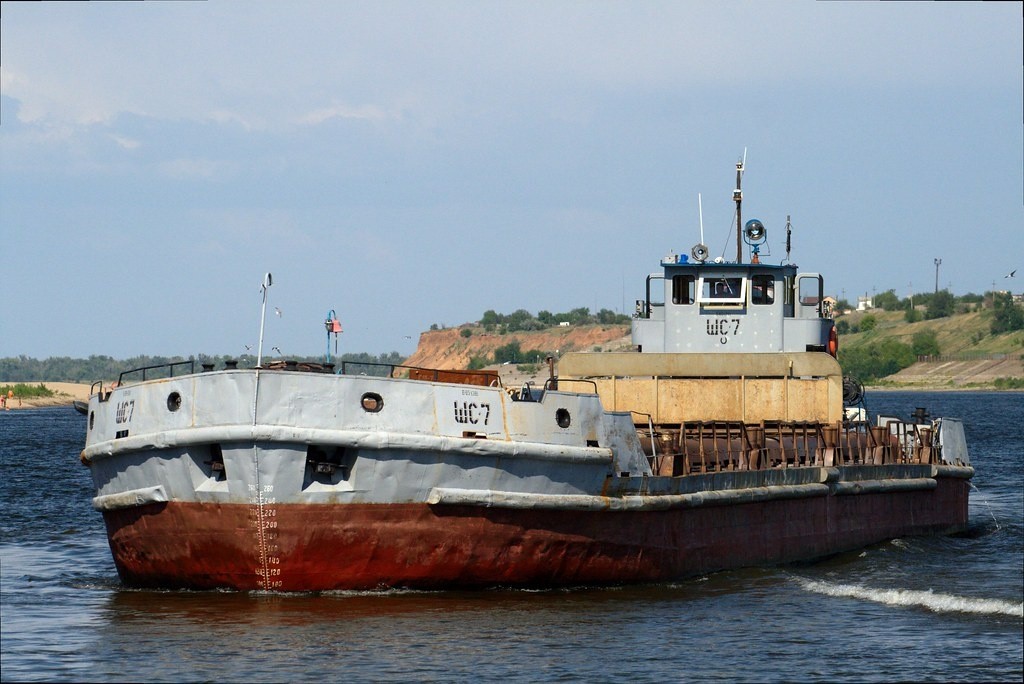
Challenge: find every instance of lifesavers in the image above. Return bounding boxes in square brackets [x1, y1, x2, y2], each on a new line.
[828, 323, 840, 359]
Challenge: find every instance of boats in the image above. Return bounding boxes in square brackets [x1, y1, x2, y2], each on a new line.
[79, 147, 977, 597]
[72, 401, 88, 416]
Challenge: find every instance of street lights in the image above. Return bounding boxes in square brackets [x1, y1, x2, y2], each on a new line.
[933, 259, 944, 294]
